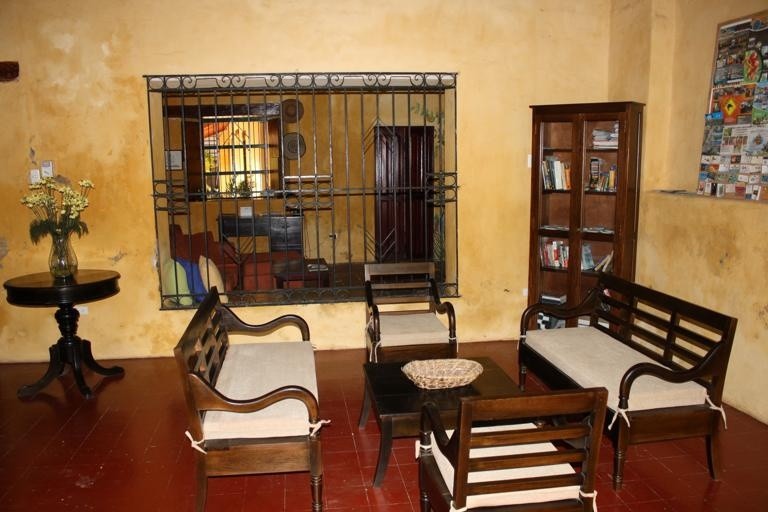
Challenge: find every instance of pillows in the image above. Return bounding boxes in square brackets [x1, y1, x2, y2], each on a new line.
[174, 256, 207, 305]
[199, 256, 230, 305]
[162, 258, 192, 307]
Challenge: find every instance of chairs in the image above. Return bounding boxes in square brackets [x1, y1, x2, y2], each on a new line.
[418, 386, 608, 512]
[363, 261, 457, 365]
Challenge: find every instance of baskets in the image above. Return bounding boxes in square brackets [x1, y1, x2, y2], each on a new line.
[400, 359, 484, 389]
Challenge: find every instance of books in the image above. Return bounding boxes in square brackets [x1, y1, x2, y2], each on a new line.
[532, 118, 623, 330]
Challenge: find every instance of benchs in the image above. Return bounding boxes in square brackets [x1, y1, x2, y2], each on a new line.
[173, 286, 324, 512]
[518, 271, 738, 491]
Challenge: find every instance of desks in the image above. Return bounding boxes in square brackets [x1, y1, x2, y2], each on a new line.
[277, 255, 332, 287]
[217, 212, 303, 260]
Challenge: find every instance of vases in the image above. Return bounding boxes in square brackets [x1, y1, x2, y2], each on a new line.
[48, 229, 78, 278]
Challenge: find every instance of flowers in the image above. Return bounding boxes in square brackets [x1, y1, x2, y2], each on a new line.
[19, 173, 96, 272]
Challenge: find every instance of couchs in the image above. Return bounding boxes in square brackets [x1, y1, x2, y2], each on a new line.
[169, 224, 238, 290]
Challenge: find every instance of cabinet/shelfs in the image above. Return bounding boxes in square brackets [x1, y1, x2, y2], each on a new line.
[526, 101, 647, 341]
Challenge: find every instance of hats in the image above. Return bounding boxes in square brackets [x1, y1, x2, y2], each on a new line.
[283, 132, 306, 160]
[282, 99, 304, 123]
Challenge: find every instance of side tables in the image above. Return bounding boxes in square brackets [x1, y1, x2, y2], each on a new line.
[3, 269, 121, 401]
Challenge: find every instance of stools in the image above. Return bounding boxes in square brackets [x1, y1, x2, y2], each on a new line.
[240, 250, 305, 290]
